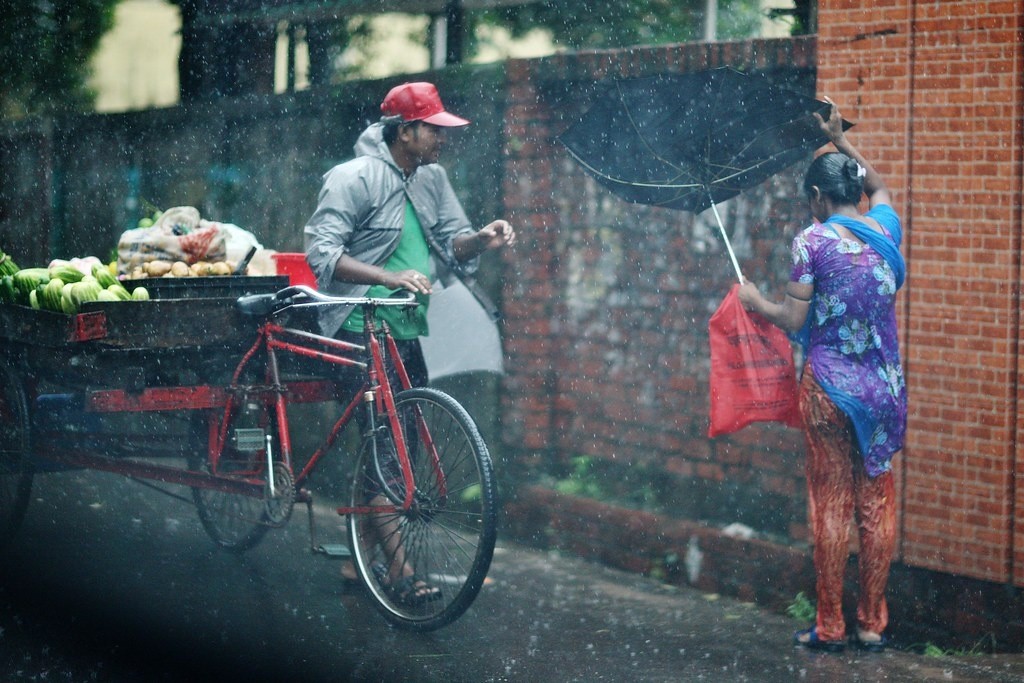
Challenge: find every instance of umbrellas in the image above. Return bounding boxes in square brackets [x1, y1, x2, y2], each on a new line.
[551, 64, 857, 312]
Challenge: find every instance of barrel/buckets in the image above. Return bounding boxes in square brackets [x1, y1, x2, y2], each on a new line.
[272, 253, 318, 292]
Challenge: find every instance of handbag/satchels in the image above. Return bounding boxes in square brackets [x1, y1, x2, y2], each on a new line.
[708, 282, 802, 439]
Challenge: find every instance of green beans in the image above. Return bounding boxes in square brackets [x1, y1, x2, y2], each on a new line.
[0, 248, 22, 279]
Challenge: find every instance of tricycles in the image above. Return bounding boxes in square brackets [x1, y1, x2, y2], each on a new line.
[0, 254, 501, 631]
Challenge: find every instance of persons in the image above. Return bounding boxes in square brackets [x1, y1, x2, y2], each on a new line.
[303, 82, 518, 606]
[738, 96, 909, 652]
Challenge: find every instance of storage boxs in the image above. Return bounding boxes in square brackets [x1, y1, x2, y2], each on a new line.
[271, 253, 318, 290]
[121, 276, 290, 298]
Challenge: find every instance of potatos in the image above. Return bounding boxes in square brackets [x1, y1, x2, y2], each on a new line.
[117, 232, 240, 280]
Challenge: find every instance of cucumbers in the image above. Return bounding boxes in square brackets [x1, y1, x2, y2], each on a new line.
[0, 264, 150, 312]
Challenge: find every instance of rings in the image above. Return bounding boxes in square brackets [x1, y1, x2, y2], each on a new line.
[413, 274, 419, 279]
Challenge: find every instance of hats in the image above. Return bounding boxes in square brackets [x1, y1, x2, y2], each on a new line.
[380, 81, 472, 127]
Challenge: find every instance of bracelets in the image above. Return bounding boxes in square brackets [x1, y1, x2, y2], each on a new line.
[787, 292, 812, 302]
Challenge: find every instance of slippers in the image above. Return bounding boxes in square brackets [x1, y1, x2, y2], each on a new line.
[344, 564, 388, 587]
[384, 575, 442, 606]
[795, 623, 845, 652]
[848, 624, 887, 651]
